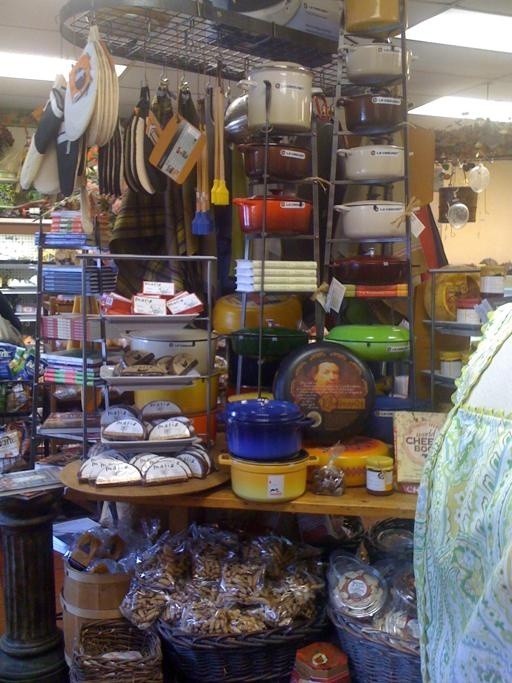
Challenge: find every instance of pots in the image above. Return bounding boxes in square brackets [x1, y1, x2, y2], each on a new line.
[325, 245, 409, 285]
[234, 188, 316, 234]
[214, 396, 317, 460]
[230, 320, 305, 358]
[336, 139, 406, 181]
[326, 318, 407, 362]
[342, 1, 405, 38]
[338, 86, 406, 131]
[215, 291, 304, 332]
[218, 447, 317, 502]
[370, 388, 406, 438]
[336, 38, 404, 84]
[239, 138, 315, 181]
[332, 194, 407, 237]
[120, 329, 225, 448]
[303, 436, 389, 486]
[274, 337, 375, 440]
[236, 61, 322, 135]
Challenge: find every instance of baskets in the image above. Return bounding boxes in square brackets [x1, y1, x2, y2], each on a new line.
[369, 518, 414, 560]
[317, 520, 363, 563]
[156, 599, 325, 680]
[71, 618, 162, 680]
[326, 606, 420, 680]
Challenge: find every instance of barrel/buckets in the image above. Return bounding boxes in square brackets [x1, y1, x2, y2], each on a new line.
[58, 556, 132, 667]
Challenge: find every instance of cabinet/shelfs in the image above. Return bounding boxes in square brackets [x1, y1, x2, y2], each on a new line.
[0, 2, 484, 470]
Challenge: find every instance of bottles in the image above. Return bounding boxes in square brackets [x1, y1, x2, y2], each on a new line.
[364, 457, 395, 496]
[439, 350, 462, 379]
[454, 265, 512, 326]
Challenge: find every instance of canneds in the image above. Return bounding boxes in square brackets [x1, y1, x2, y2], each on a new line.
[364, 455, 395, 496]
